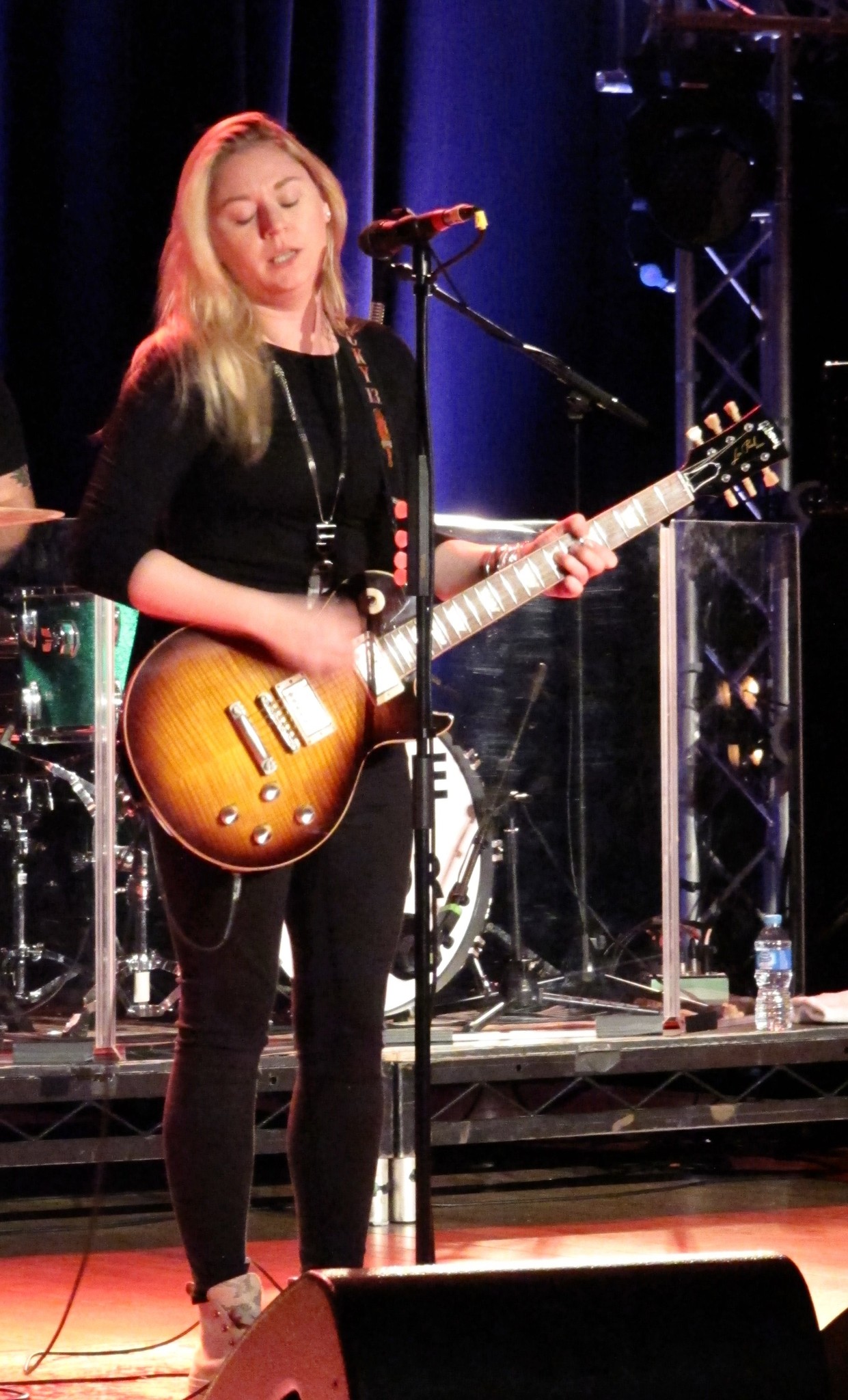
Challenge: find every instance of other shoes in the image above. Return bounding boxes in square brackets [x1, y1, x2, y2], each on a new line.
[185, 1272, 262, 1393]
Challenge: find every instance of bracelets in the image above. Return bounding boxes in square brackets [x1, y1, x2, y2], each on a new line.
[481, 546, 521, 580]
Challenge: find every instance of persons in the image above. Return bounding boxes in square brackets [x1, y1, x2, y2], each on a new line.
[78, 113, 619, 1389]
[0, 379, 35, 577]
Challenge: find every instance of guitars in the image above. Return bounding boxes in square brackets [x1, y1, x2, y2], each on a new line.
[121, 402, 791, 871]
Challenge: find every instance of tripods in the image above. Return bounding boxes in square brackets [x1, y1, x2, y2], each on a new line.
[395, 242, 722, 1034]
[0, 725, 186, 1038]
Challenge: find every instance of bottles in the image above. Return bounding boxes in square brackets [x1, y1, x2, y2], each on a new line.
[753, 914, 796, 1031]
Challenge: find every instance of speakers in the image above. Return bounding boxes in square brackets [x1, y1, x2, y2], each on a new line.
[202, 1250, 848, 1399]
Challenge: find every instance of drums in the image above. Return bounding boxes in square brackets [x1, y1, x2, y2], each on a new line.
[12, 583, 138, 746]
[278, 730, 492, 1017]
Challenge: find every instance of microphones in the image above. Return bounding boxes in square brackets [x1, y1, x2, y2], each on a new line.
[357, 204, 477, 260]
[370, 256, 389, 325]
[397, 937, 443, 981]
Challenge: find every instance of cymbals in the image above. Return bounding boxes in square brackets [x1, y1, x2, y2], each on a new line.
[0, 506, 64, 528]
